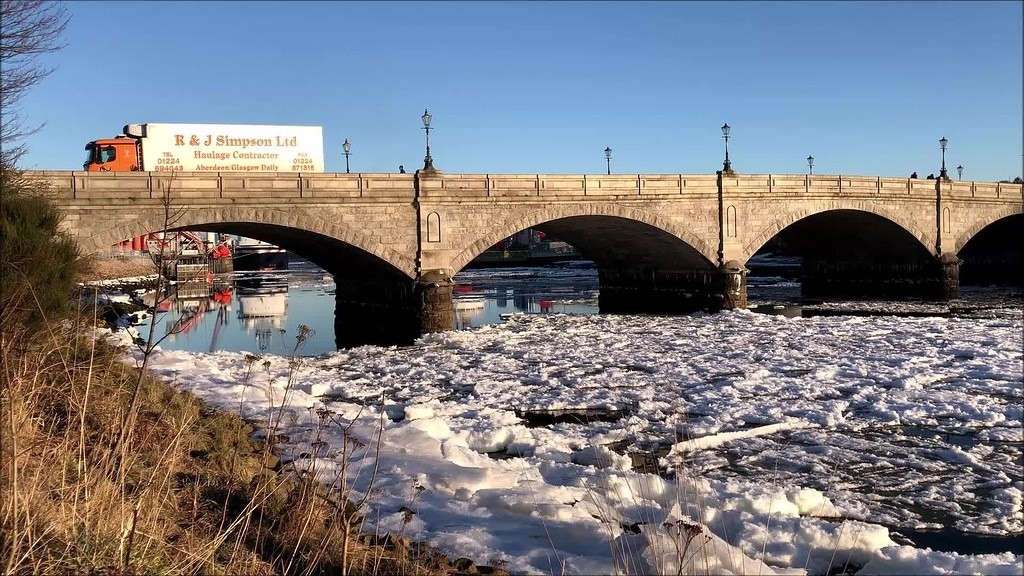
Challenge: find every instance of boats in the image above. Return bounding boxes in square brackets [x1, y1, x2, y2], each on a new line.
[231, 236, 289, 269]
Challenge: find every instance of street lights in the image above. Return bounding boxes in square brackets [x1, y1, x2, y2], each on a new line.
[419, 109, 435, 171]
[341, 138, 352, 173]
[940, 136, 948, 176]
[604, 146, 613, 175]
[807, 155, 814, 174]
[957, 165, 963, 181]
[721, 123, 734, 171]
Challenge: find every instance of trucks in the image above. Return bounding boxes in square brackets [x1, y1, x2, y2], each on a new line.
[83, 121, 325, 173]
